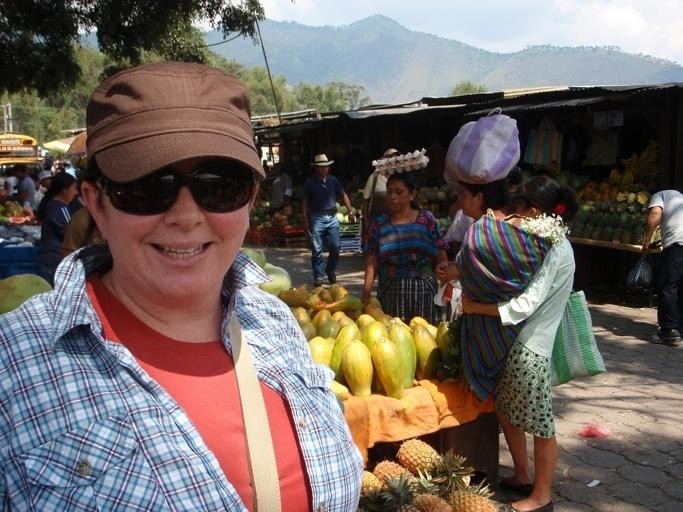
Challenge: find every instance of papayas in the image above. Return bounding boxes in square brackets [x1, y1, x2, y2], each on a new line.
[268, 284, 454, 399]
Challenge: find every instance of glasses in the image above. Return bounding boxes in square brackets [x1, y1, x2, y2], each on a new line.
[106, 159, 254, 216]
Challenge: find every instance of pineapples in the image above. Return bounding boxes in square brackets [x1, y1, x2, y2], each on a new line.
[565, 210, 645, 245]
[361, 438, 498, 512]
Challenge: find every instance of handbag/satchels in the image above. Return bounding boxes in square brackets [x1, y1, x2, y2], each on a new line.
[548, 290, 606, 387]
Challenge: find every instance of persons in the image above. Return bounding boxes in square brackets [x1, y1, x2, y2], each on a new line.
[365, 149, 400, 218]
[366, 171, 447, 327]
[303, 154, 355, 288]
[262, 159, 268, 172]
[271, 158, 291, 212]
[0, 62, 364, 511]
[436, 165, 577, 512]
[0, 160, 109, 290]
[639, 173, 682, 345]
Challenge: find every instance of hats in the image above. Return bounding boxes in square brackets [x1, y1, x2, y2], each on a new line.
[310, 154, 335, 166]
[85, 61, 267, 183]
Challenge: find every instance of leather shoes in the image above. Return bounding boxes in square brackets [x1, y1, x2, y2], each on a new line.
[511, 500, 554, 512]
[499, 476, 533, 496]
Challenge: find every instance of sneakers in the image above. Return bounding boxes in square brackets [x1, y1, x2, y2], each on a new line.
[313, 266, 336, 287]
[650, 328, 680, 346]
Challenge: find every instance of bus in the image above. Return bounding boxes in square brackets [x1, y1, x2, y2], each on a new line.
[0, 130, 45, 156]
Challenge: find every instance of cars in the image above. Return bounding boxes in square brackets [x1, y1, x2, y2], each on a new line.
[0, 157, 49, 182]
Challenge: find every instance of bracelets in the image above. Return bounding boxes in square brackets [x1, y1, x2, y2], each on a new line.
[348, 211, 352, 215]
